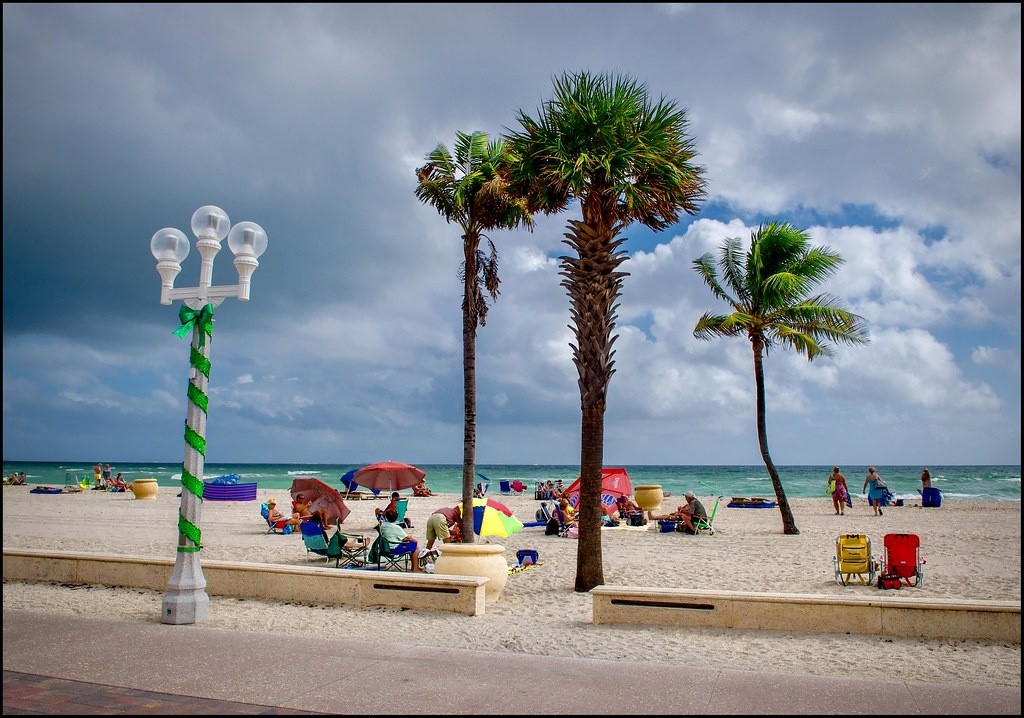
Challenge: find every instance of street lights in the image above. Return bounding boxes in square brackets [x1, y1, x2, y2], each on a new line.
[150, 205, 268, 626]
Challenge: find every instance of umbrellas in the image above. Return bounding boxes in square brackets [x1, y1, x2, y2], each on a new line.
[559, 468, 631, 514]
[459, 498, 524, 543]
[290, 477, 351, 523]
[354, 460, 427, 501]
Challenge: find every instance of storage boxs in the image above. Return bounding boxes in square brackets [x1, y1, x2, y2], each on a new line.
[878, 576, 901, 589]
[516, 549, 538, 564]
[659, 520, 676, 533]
[626, 513, 643, 526]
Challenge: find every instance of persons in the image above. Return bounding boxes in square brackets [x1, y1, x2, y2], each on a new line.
[424, 507, 462, 562]
[380, 509, 423, 572]
[375, 492, 400, 524]
[8, 472, 25, 484]
[560, 493, 579, 528]
[883, 481, 892, 505]
[863, 467, 883, 515]
[540, 479, 563, 500]
[292, 494, 332, 529]
[302, 515, 371, 548]
[94, 463, 133, 490]
[828, 467, 847, 515]
[921, 468, 931, 489]
[648, 492, 708, 535]
[267, 498, 300, 526]
[413, 480, 434, 497]
[617, 494, 647, 524]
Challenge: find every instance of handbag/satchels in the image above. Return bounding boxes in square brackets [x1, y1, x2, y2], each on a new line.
[874, 478, 887, 489]
[826, 475, 836, 493]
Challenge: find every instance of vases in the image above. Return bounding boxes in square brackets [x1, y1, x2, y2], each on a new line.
[134, 479, 159, 500]
[435, 543, 508, 604]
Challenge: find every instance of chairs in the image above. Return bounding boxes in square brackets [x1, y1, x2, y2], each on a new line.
[104, 479, 125, 492]
[540, 502, 562, 527]
[260, 502, 281, 535]
[879, 533, 926, 589]
[335, 517, 369, 568]
[377, 520, 411, 572]
[554, 503, 574, 537]
[832, 534, 878, 589]
[499, 481, 512, 495]
[411, 487, 425, 496]
[478, 484, 489, 498]
[13, 474, 27, 485]
[512, 482, 525, 496]
[300, 521, 328, 563]
[291, 500, 313, 523]
[693, 501, 719, 535]
[375, 497, 408, 529]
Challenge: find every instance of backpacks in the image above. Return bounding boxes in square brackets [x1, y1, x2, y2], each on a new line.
[545, 518, 559, 534]
